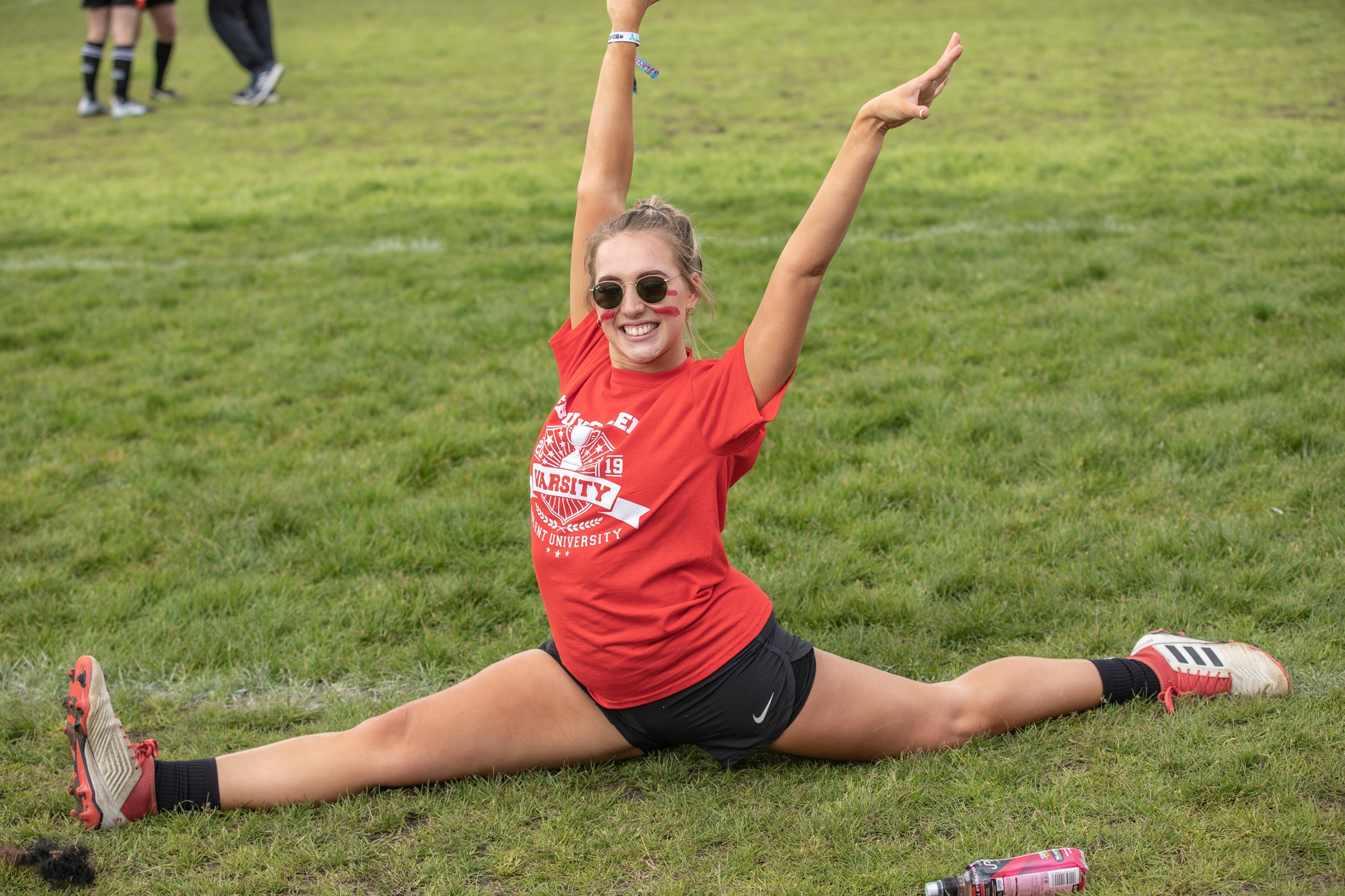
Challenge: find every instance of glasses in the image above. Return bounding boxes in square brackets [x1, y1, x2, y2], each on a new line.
[589, 273, 684, 311]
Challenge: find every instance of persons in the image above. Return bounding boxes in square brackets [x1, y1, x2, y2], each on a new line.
[207, 1, 286, 108]
[61, 0, 1291, 831]
[77, 0, 179, 119]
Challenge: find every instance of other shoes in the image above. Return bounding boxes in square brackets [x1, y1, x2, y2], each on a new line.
[231, 61, 285, 107]
[110, 97, 147, 117]
[149, 86, 177, 102]
[76, 98, 101, 116]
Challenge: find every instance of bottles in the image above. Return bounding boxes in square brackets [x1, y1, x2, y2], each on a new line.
[925, 848, 1088, 896]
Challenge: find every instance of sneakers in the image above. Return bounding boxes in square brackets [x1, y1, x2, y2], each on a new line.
[62, 655, 158, 828]
[1122, 627, 1291, 714]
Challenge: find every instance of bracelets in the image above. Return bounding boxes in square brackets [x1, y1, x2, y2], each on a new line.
[607, 31, 660, 95]
